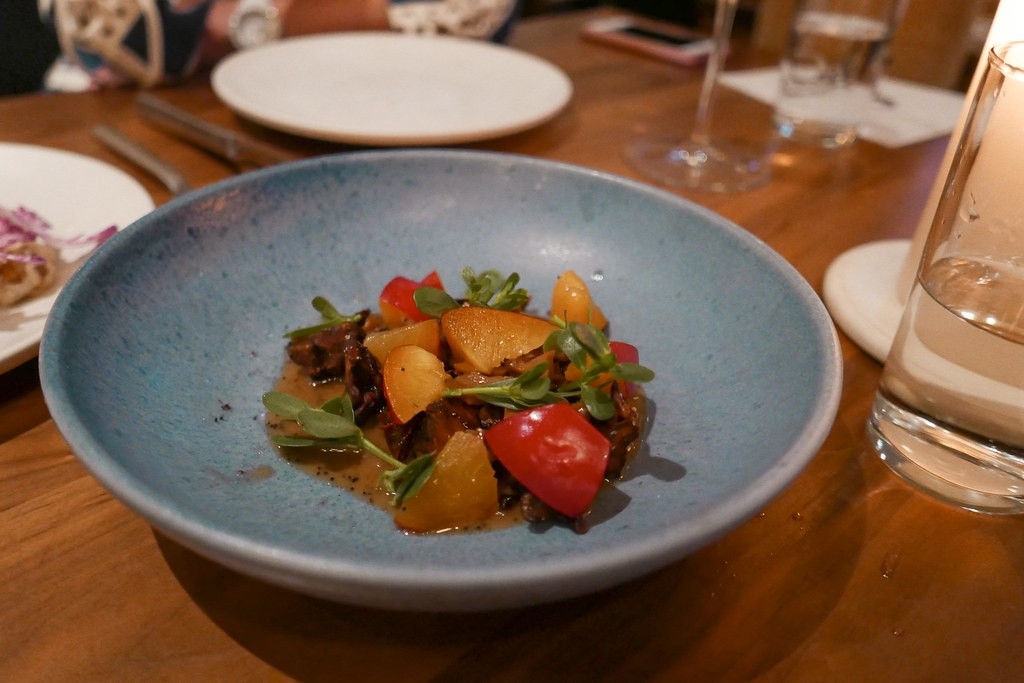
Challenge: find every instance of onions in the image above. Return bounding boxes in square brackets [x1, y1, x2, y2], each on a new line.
[0, 206, 120, 266]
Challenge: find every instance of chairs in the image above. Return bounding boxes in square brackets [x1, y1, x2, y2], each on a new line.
[751, 0, 976, 88]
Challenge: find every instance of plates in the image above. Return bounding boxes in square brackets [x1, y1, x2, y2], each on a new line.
[0, 141, 157, 378]
[37, 149, 843, 613]
[210, 33, 574, 146]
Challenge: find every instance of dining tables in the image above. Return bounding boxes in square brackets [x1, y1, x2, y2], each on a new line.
[0, 9, 1024, 683]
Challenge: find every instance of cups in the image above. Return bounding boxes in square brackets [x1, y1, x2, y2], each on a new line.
[770, 0, 898, 148]
[866, 49, 1023, 515]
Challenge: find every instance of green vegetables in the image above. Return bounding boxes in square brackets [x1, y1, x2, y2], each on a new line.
[263, 265, 653, 506]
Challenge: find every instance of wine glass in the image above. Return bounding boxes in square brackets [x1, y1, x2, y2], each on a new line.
[623, 1, 773, 195]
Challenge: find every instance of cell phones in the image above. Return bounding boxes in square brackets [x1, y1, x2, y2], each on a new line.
[580, 16, 715, 62]
